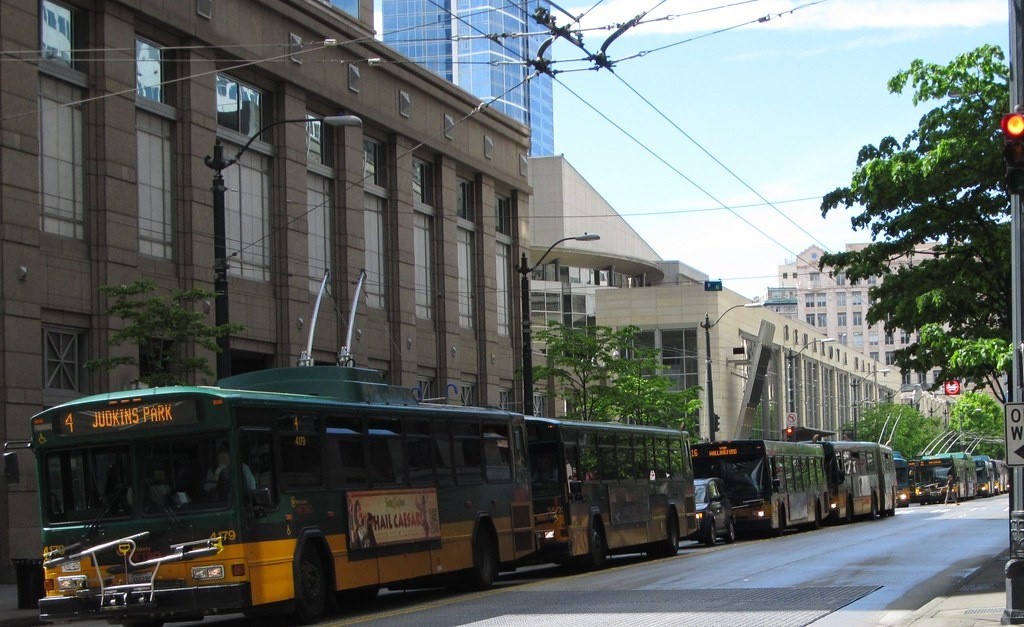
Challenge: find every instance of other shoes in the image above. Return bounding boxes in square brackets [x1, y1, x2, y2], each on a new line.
[956, 504, 960, 506]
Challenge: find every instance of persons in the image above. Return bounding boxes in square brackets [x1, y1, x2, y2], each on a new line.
[203, 447, 256, 507]
[944, 475, 960, 506]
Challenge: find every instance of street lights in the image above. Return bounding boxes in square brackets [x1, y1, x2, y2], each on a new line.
[204, 112, 364, 383]
[849, 369, 891, 442]
[929, 400, 958, 425]
[958, 408, 982, 452]
[511, 231, 599, 416]
[943, 403, 969, 427]
[698, 302, 763, 439]
[883, 384, 921, 415]
[786, 338, 837, 443]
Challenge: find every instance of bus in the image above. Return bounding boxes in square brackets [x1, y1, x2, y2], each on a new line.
[893, 450, 1009, 507]
[691, 438, 900, 538]
[0, 359, 698, 627]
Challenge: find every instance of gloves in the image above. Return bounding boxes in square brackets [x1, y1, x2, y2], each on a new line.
[215, 463, 228, 476]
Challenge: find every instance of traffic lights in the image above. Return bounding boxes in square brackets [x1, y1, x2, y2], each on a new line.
[999, 110, 1023, 196]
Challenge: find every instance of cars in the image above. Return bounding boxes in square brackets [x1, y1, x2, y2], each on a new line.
[694, 478, 736, 545]
